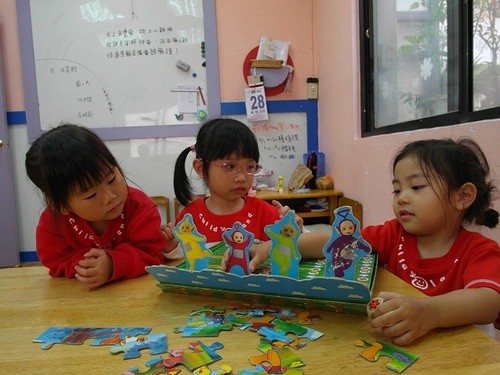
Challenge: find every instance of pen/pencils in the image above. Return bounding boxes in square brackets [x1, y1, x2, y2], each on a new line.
[198, 86, 206, 105]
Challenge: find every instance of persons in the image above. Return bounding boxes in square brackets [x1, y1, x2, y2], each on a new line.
[159, 119, 305, 275]
[24, 120, 168, 288]
[221, 137, 500, 350]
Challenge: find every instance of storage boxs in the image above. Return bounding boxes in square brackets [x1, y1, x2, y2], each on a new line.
[156, 240, 380, 317]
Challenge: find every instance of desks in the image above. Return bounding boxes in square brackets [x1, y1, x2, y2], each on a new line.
[0, 258, 500, 375]
[255, 189, 343, 225]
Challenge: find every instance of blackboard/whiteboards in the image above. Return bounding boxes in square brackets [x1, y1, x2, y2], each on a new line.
[6, 99, 318, 265]
[28, 0, 209, 131]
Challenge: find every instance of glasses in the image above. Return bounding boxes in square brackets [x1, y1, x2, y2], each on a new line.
[210, 162, 262, 176]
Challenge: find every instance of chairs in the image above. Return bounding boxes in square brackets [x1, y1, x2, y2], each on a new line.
[174, 194, 205, 221]
[149, 195, 170, 226]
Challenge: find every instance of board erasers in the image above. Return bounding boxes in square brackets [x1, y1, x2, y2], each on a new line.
[176, 59, 190, 72]
